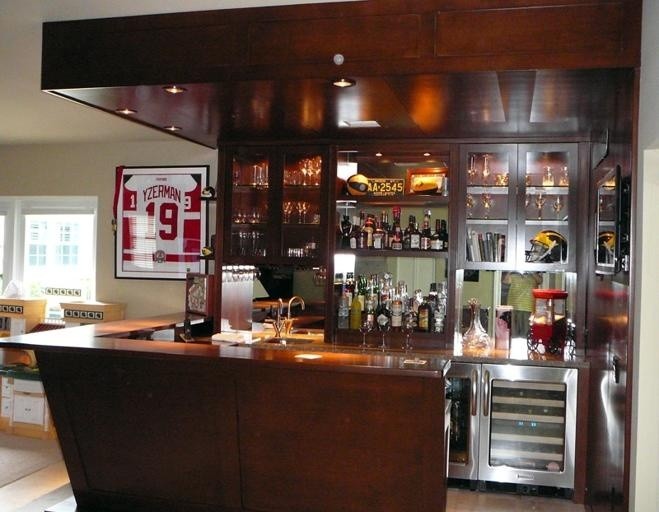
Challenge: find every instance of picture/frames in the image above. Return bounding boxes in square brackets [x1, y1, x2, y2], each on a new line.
[113, 165, 209, 281]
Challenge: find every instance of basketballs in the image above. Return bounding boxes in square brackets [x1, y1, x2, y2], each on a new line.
[346, 174, 369, 196]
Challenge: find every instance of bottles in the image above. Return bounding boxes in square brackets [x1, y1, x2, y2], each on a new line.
[543, 164, 571, 186]
[461, 299, 494, 355]
[335, 205, 447, 334]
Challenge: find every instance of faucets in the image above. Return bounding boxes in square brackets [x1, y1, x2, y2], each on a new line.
[285, 295, 307, 337]
[265, 298, 285, 337]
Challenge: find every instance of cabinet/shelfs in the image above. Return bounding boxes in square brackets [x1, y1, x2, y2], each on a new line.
[220, 141, 581, 351]
[448, 361, 580, 493]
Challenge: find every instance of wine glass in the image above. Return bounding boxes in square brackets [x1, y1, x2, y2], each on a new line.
[466, 191, 563, 221]
[359, 315, 415, 352]
[283, 156, 321, 223]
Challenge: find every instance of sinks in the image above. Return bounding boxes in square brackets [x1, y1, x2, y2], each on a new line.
[290, 327, 326, 337]
[251, 335, 315, 346]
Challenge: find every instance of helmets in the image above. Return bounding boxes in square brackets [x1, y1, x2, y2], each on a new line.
[525, 229, 567, 263]
[599, 231, 616, 247]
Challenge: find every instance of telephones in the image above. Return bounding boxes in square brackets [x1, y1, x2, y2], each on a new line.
[180, 320, 195, 343]
